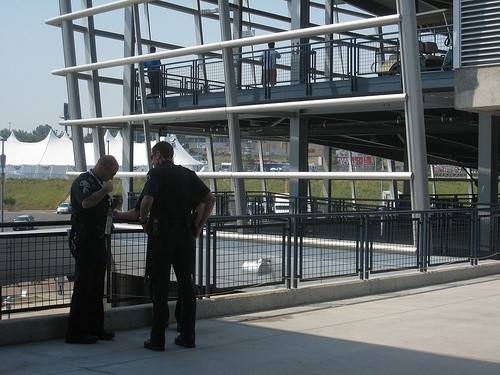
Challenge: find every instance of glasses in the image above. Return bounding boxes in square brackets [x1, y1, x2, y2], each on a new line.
[151, 153, 156, 160]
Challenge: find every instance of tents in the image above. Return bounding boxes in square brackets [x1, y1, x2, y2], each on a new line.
[0, 129, 207, 178]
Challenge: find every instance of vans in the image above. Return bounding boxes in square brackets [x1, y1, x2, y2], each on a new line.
[274, 193, 289, 214]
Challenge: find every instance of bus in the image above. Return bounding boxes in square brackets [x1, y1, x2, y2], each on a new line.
[255, 162, 316, 171]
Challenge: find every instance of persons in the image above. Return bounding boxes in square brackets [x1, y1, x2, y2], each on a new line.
[54, 271, 65, 295]
[143, 47, 162, 98]
[65, 155, 119, 344]
[260, 43, 281, 87]
[113, 189, 205, 332]
[139, 142, 215, 351]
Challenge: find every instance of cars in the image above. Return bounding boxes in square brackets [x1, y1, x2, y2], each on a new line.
[56, 202, 71, 213]
[248, 201, 261, 218]
[13, 213, 33, 229]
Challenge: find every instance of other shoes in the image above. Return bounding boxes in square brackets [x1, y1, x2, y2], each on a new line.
[144, 340, 165, 351]
[92, 330, 115, 340]
[174, 336, 195, 348]
[65, 333, 97, 343]
